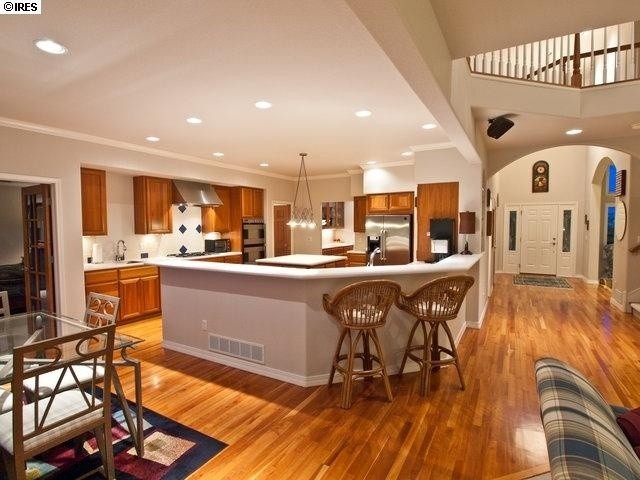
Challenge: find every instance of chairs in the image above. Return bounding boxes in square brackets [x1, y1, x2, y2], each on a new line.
[317, 276, 402, 410]
[533, 356, 640, 480]
[394, 273, 477, 398]
[0, 291, 40, 416]
[0, 322, 121, 480]
[22, 290, 125, 454]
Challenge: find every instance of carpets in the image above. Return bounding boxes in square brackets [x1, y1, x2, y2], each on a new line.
[23, 382, 231, 480]
[512, 271, 572, 289]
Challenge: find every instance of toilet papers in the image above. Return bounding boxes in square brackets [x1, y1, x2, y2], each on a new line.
[92, 244, 103, 263]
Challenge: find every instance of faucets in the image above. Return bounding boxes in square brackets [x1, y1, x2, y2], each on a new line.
[368, 247, 381, 265]
[113, 239, 127, 261]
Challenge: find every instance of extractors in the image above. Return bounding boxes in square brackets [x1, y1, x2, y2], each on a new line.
[172, 179, 224, 206]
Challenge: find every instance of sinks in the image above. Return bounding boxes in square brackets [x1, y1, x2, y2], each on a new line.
[112, 259, 144, 268]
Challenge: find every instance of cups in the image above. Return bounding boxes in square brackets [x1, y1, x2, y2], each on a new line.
[88, 257, 91, 263]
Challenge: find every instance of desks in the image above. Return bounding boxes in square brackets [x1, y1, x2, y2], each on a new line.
[253, 252, 349, 270]
[0, 308, 147, 464]
[139, 251, 484, 389]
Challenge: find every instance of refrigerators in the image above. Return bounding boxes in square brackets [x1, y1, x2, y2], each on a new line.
[364, 214, 411, 266]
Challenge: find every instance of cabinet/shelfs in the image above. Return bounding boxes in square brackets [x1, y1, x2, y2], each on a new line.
[131, 175, 174, 236]
[366, 190, 414, 215]
[80, 168, 108, 238]
[322, 201, 345, 231]
[353, 195, 366, 234]
[348, 253, 366, 267]
[231, 186, 264, 251]
[322, 244, 354, 268]
[85, 268, 121, 329]
[200, 254, 244, 265]
[119, 265, 160, 324]
[200, 186, 231, 235]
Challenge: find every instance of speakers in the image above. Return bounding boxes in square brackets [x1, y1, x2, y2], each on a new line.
[487, 118, 515, 139]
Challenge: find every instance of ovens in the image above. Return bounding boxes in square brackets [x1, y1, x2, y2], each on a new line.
[242, 218, 266, 264]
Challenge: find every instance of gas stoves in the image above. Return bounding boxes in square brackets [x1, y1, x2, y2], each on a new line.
[166, 252, 207, 257]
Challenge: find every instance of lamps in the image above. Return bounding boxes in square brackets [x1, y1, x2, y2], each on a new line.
[457, 209, 476, 255]
[284, 153, 319, 230]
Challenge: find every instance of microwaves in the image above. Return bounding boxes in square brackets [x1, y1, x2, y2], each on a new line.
[205, 239, 230, 252]
